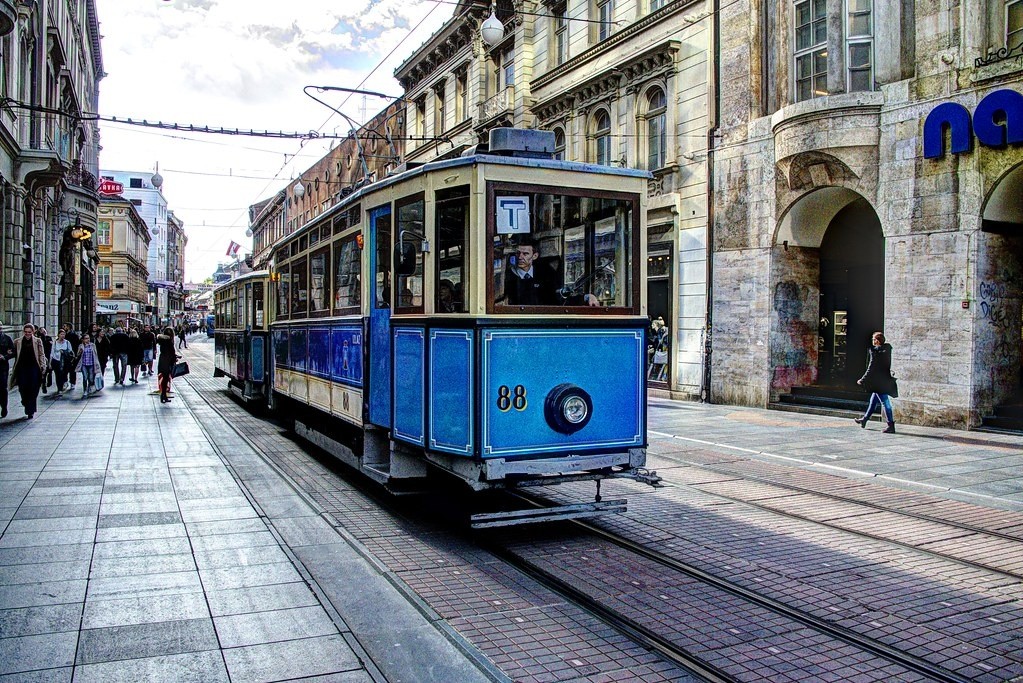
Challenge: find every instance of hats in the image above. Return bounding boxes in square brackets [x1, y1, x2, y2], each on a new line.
[439, 279, 456, 292]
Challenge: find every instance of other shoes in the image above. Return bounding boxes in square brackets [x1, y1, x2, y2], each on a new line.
[71, 383, 75, 390]
[142, 371, 154, 377]
[120, 381, 123, 384]
[185, 347, 187, 348]
[179, 348, 181, 349]
[135, 380, 138, 383]
[43, 387, 47, 393]
[1, 408, 8, 417]
[129, 378, 134, 381]
[63, 382, 69, 389]
[28, 414, 33, 419]
[116, 381, 119, 383]
[58, 389, 63, 396]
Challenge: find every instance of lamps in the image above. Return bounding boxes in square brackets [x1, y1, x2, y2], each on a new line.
[294, 181, 304, 197]
[152, 170, 163, 187]
[67, 207, 84, 239]
[232, 253, 237, 259]
[246, 228, 253, 236]
[174, 270, 178, 276]
[152, 226, 160, 235]
[481, 11, 504, 45]
[159, 250, 165, 257]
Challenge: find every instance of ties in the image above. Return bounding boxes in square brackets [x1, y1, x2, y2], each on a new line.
[524, 273, 531, 279]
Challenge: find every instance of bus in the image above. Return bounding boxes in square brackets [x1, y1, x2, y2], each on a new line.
[206, 314, 216, 338]
[212, 269, 273, 405]
[263, 126, 669, 530]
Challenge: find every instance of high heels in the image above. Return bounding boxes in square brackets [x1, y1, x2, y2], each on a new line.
[160, 394, 171, 402]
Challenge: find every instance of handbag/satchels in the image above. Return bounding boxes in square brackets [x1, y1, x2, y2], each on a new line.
[95, 373, 103, 391]
[888, 376, 898, 398]
[171, 362, 189, 378]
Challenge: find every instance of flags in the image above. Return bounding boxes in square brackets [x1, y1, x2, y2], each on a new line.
[226, 242, 240, 256]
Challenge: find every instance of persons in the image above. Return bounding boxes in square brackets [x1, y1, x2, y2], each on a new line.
[495, 238, 600, 307]
[855, 332, 898, 433]
[381, 288, 413, 308]
[0, 321, 205, 418]
[439, 279, 460, 313]
[647, 318, 668, 381]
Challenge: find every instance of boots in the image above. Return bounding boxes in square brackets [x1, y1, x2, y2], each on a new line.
[881, 421, 896, 433]
[854, 415, 868, 428]
